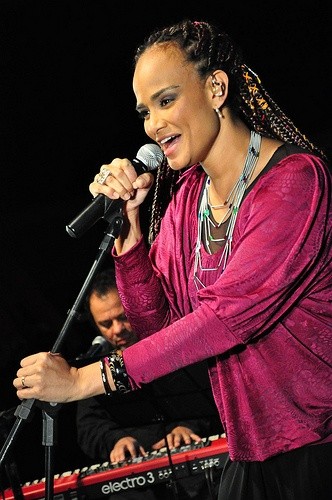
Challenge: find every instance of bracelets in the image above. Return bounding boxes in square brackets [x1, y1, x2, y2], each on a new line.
[99, 352, 132, 395]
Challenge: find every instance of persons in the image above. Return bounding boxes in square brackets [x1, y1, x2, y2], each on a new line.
[12, 18, 332, 500]
[76, 270, 211, 500]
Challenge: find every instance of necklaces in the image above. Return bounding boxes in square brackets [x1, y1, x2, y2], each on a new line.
[194, 131, 261, 291]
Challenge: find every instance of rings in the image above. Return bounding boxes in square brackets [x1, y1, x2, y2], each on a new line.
[22, 376, 26, 387]
[97, 169, 111, 184]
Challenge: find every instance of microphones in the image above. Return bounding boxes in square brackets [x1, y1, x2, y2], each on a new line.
[86, 336, 110, 357]
[66, 144, 164, 239]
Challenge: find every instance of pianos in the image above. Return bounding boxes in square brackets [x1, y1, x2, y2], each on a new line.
[0, 433, 232, 500]
